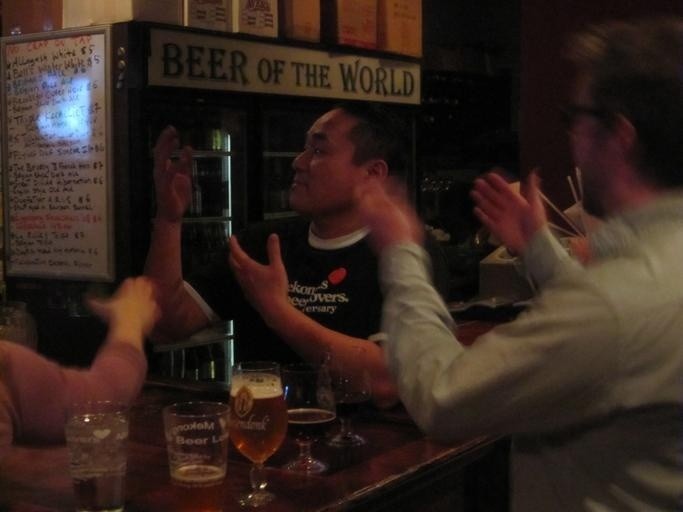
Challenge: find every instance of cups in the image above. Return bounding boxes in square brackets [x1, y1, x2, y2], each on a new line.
[161, 400, 232, 490]
[63, 399, 134, 512]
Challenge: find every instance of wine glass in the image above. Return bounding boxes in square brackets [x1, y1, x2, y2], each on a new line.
[227, 371, 289, 508]
[317, 345, 376, 448]
[285, 407, 337, 474]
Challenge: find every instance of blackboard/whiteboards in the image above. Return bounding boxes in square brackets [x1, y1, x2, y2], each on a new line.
[0, 24, 114, 282]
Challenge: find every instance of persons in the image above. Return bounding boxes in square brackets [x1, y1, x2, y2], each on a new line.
[351, 15, 683, 511]
[0, 275, 158, 511]
[149, 106, 411, 404]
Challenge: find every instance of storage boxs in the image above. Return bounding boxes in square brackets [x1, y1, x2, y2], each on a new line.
[62, 0, 423, 58]
